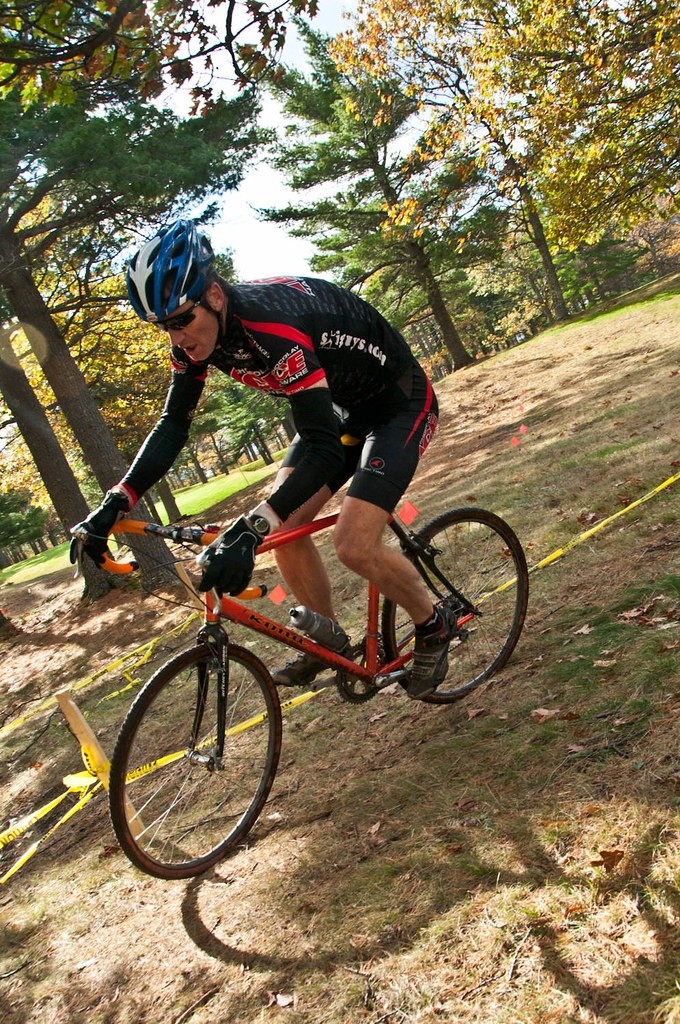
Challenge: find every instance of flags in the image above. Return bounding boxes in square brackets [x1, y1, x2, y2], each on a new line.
[268, 582, 287, 605]
[511, 437, 520, 448]
[395, 501, 421, 527]
[519, 424, 528, 435]
[520, 406, 524, 412]
[522, 389, 525, 396]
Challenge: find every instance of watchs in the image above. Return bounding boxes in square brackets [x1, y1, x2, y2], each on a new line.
[245, 510, 272, 538]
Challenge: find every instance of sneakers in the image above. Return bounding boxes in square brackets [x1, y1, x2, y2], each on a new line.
[407, 606, 458, 700]
[269, 652, 332, 687]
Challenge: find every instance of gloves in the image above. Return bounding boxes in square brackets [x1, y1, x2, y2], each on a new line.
[196, 513, 265, 599]
[69, 486, 130, 570]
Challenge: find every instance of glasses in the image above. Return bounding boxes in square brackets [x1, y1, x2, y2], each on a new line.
[152, 297, 206, 332]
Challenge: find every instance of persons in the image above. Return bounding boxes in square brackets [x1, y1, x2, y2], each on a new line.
[70, 215, 458, 701]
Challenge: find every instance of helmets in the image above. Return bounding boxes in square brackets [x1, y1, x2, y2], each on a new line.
[126, 218, 216, 324]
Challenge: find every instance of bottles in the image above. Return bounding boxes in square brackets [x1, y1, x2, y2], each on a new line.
[289, 605, 348, 650]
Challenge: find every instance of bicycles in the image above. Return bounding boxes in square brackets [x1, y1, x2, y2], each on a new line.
[70, 435, 532, 882]
